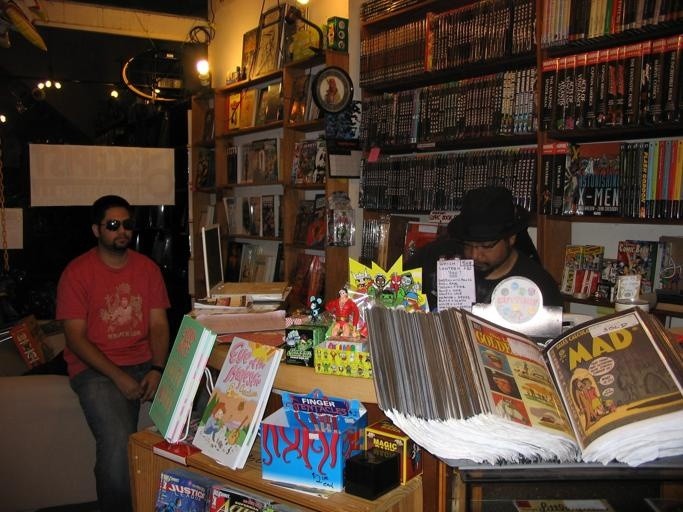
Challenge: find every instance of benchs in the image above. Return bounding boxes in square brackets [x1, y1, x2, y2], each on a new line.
[0, 375, 155, 511]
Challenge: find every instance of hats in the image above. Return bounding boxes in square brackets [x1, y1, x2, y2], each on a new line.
[447, 187, 529, 242]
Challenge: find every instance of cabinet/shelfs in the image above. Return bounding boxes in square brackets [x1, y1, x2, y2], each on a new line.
[189, 310, 453, 512]
[191, 49, 349, 303]
[127, 425, 424, 512]
[363, 0, 683, 313]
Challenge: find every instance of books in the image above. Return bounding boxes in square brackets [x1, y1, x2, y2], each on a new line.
[144, 1, 682, 511]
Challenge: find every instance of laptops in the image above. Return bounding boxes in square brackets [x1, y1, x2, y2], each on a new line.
[201, 224, 289, 302]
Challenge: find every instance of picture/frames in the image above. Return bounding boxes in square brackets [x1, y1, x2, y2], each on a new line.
[312, 66, 354, 113]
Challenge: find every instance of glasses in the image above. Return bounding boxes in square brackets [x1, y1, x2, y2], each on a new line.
[463, 240, 500, 251]
[101, 218, 133, 231]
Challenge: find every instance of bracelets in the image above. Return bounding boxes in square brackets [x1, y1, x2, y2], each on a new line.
[149, 364, 164, 374]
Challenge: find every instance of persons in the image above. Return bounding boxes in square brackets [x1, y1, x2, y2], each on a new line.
[54, 194, 171, 511]
